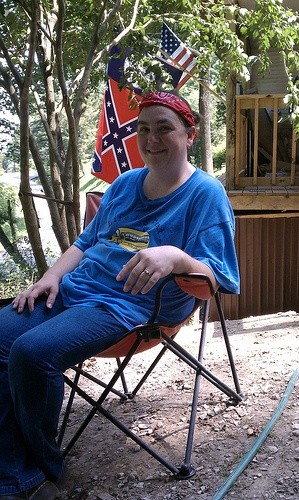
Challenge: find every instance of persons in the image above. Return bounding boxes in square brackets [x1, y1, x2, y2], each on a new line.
[1, 91, 240, 499]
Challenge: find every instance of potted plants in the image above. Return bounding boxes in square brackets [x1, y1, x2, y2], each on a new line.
[240, 0, 299, 94]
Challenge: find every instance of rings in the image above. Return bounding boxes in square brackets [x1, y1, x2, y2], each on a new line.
[143, 270, 151, 278]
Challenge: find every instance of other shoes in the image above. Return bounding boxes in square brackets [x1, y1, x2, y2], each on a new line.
[27, 480, 60, 500]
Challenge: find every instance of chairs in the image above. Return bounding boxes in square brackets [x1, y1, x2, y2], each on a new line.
[57, 191, 244, 480]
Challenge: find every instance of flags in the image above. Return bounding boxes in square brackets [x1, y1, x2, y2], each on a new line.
[90, 24, 183, 184]
[140, 14, 199, 92]
